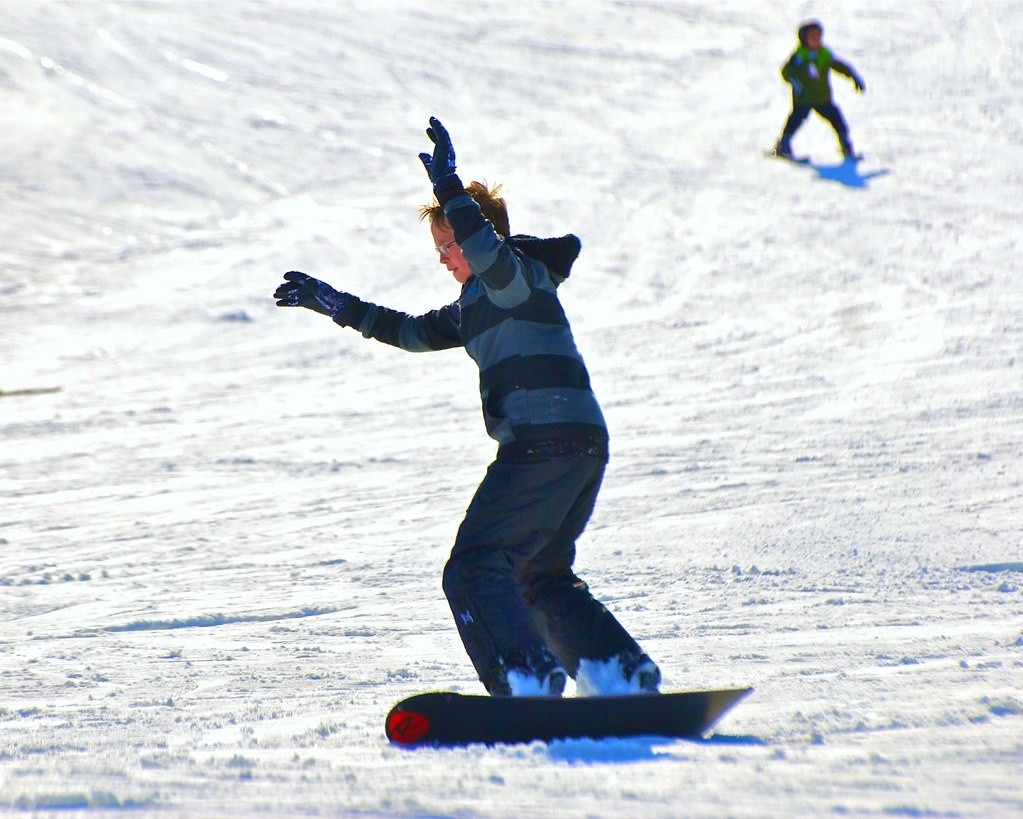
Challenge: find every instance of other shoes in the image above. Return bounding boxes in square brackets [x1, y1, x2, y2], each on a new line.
[486, 648, 566, 696]
[622, 654, 661, 693]
[842, 144, 854, 158]
[776, 141, 791, 155]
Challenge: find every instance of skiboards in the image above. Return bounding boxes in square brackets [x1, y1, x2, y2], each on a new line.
[763, 148, 866, 188]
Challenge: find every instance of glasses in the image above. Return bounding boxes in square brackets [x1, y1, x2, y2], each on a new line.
[433, 240, 455, 257]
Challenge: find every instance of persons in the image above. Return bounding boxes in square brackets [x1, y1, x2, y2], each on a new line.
[272, 115, 663, 698]
[774, 22, 865, 162]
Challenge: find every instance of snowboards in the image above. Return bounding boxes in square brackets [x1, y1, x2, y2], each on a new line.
[384, 685, 756, 751]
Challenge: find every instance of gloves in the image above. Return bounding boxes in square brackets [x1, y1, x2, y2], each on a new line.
[273, 271, 360, 328]
[852, 73, 865, 90]
[418, 116, 462, 195]
[790, 76, 803, 97]
